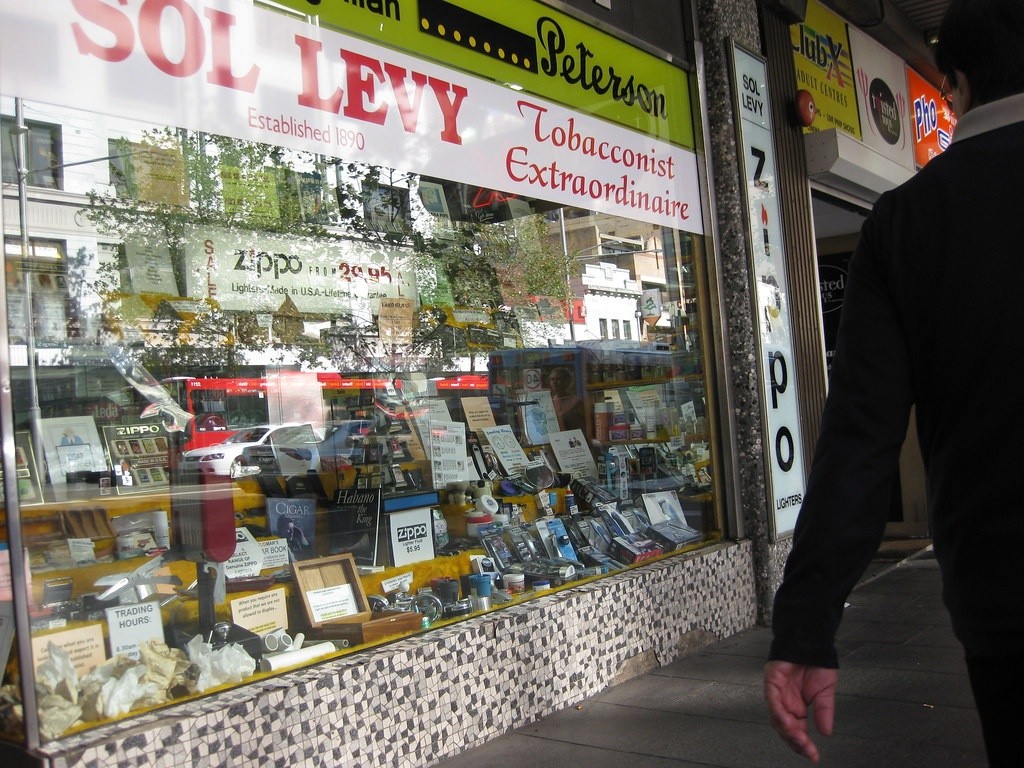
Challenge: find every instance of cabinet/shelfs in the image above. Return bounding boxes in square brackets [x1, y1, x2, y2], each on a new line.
[346, 373, 414, 495]
[581, 234, 727, 539]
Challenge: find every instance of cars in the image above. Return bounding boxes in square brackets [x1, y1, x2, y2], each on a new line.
[185, 424, 326, 479]
[244, 418, 370, 491]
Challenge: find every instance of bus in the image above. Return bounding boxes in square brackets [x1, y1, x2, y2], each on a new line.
[184, 375, 490, 451]
[487, 346, 701, 444]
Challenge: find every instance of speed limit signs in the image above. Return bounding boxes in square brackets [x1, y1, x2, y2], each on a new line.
[522, 370, 540, 390]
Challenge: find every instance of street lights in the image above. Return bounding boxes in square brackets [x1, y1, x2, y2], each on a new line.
[563, 238, 621, 343]
[19, 146, 176, 495]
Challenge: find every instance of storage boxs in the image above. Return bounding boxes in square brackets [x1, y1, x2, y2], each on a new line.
[609, 533, 663, 564]
[635, 491, 689, 527]
[533, 489, 553, 517]
[348, 394, 416, 489]
[587, 554, 626, 571]
[647, 518, 702, 551]
[570, 477, 622, 510]
[594, 402, 614, 443]
[471, 557, 498, 578]
[290, 552, 422, 647]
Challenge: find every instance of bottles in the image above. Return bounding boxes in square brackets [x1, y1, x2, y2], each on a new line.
[696, 417, 705, 433]
[671, 402, 681, 436]
[565, 485, 574, 513]
[659, 402, 671, 436]
[679, 417, 686, 446]
[644, 403, 656, 439]
[686, 416, 695, 434]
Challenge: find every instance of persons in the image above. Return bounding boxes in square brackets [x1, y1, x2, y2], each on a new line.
[277, 516, 313, 560]
[550, 367, 588, 447]
[763, 0, 1023, 767]
